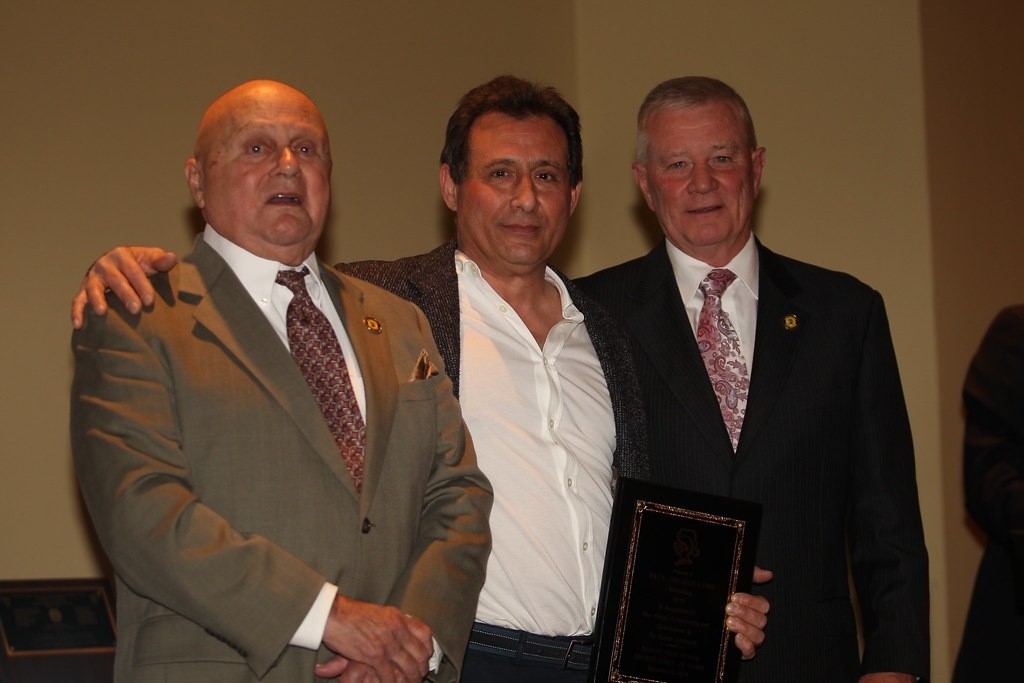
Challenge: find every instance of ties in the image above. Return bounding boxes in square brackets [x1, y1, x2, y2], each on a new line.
[274, 266, 367, 501]
[696, 268, 750, 455]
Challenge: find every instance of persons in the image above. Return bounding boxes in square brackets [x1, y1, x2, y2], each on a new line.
[567, 76, 933, 682]
[950, 304, 1024, 683]
[69, 79, 495, 682]
[68, 73, 773, 683]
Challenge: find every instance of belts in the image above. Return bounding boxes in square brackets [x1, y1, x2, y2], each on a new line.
[468, 627, 599, 674]
